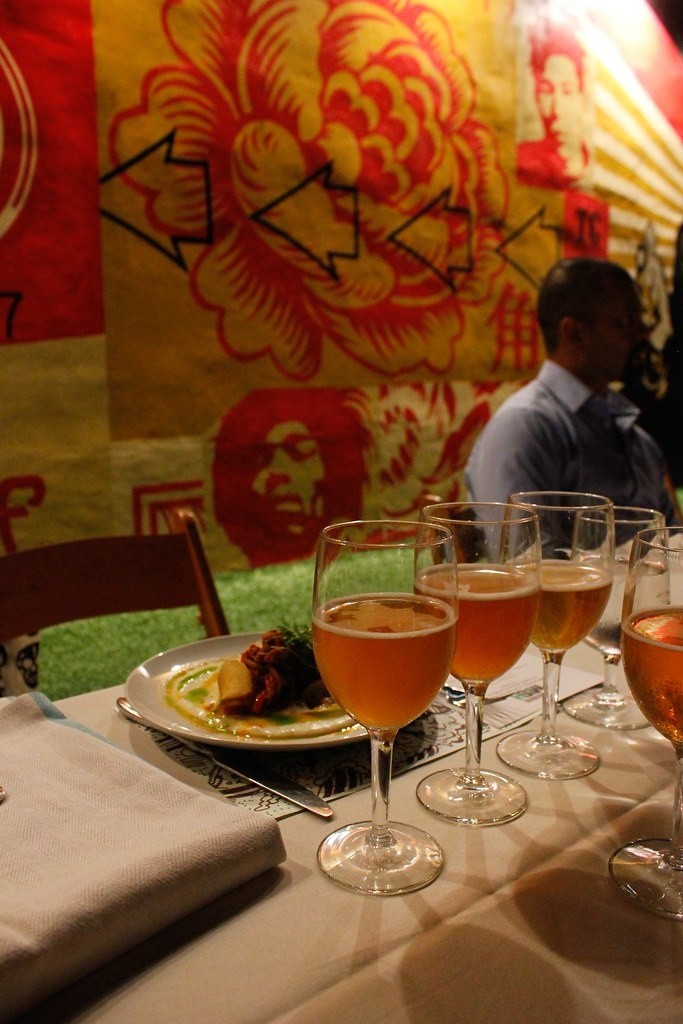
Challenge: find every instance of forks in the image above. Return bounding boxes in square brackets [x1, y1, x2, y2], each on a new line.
[441, 685, 514, 708]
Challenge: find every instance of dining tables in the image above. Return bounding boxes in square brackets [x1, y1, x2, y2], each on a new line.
[49, 535, 683, 1024]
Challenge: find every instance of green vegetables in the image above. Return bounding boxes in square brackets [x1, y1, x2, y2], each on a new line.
[272, 616, 315, 651]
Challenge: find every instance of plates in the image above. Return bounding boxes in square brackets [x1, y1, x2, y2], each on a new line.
[125, 631, 369, 750]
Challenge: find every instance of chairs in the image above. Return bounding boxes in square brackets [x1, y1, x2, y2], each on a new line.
[1, 512, 233, 645]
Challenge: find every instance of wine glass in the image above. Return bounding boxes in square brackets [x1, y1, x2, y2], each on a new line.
[311, 520, 459, 896]
[607, 527, 683, 920]
[415, 501, 544, 826]
[496, 492, 615, 778]
[562, 506, 668, 729]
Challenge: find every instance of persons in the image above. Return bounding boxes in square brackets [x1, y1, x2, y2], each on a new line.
[460, 259, 679, 553]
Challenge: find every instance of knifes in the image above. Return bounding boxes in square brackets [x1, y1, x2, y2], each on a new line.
[117, 697, 333, 818]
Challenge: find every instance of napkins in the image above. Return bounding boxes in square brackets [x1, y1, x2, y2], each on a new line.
[0, 689, 286, 1021]
